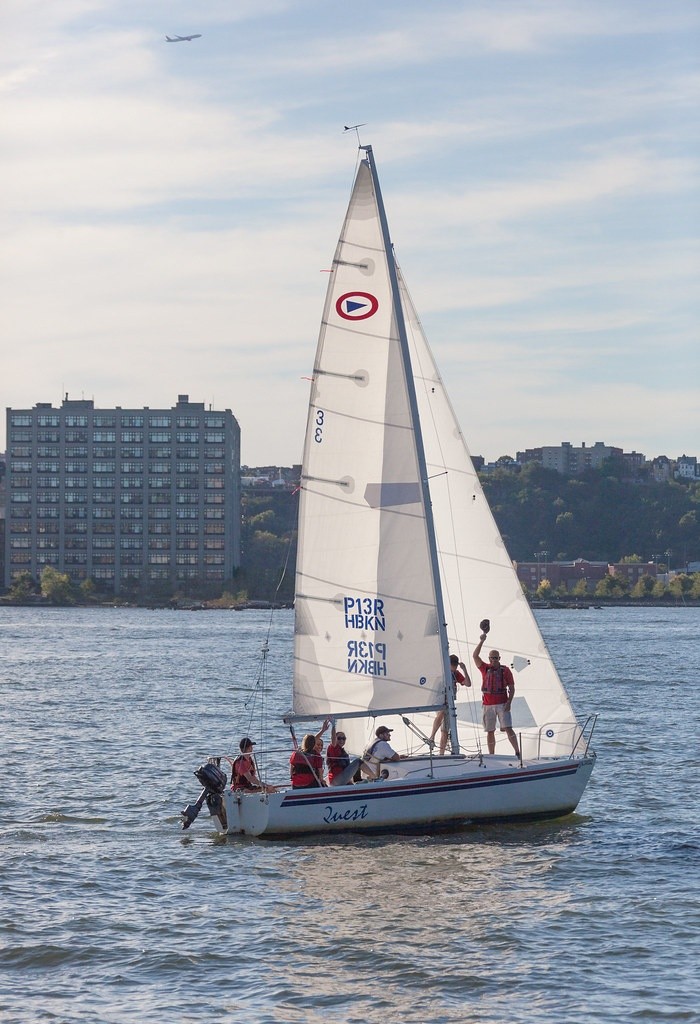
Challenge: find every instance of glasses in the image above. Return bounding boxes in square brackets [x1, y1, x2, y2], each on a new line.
[489, 657, 499, 660]
[337, 736, 346, 740]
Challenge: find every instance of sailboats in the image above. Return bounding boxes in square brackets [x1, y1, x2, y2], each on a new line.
[180, 122, 601, 839]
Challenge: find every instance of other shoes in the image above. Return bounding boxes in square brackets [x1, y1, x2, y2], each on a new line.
[516, 753, 520, 760]
[379, 774, 387, 779]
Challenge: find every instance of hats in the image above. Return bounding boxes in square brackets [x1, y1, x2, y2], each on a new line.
[240, 738, 256, 748]
[450, 655, 461, 664]
[480, 619, 489, 634]
[376, 726, 393, 736]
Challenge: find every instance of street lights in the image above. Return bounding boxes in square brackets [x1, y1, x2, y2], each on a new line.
[663, 551, 670, 574]
[652, 554, 660, 573]
[541, 550, 550, 579]
[534, 552, 541, 589]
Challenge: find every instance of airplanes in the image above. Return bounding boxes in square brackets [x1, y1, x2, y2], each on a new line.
[164, 32, 202, 42]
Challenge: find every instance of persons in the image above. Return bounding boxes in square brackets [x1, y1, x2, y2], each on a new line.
[473, 633, 522, 758]
[230, 717, 403, 794]
[430, 654, 472, 756]
